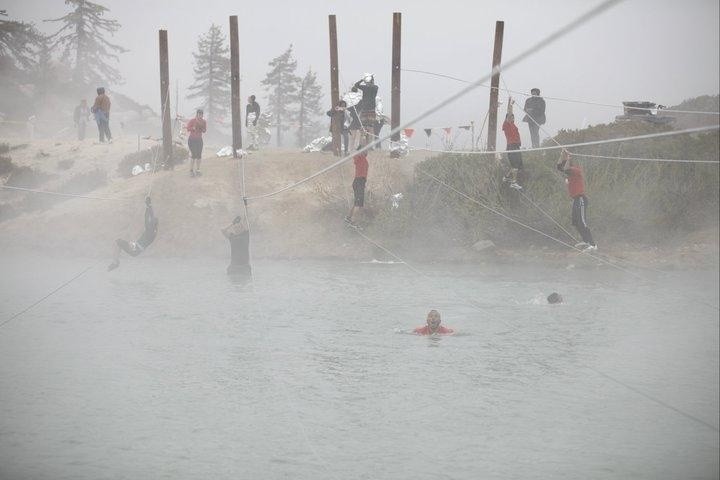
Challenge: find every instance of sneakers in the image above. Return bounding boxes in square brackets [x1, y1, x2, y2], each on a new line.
[587, 245, 597, 249]
[349, 223, 363, 230]
[504, 175, 512, 182]
[510, 183, 522, 190]
[579, 241, 590, 246]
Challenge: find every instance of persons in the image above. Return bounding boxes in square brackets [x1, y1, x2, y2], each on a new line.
[355, 75, 379, 142]
[350, 129, 370, 227]
[244, 93, 260, 151]
[525, 89, 546, 149]
[91, 87, 114, 143]
[108, 195, 158, 273]
[502, 95, 523, 190]
[546, 290, 565, 305]
[185, 110, 207, 177]
[221, 198, 252, 287]
[557, 149, 600, 253]
[414, 309, 454, 335]
[72, 98, 90, 140]
[326, 101, 351, 156]
